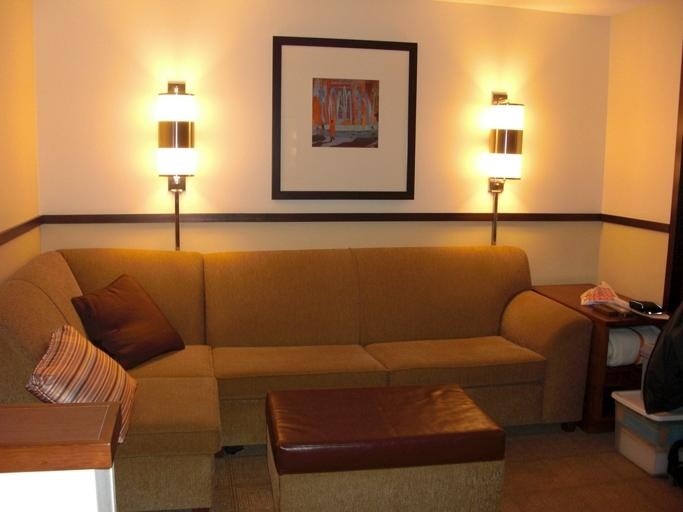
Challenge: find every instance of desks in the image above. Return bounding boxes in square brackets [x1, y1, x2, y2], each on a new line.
[529, 283, 674, 433]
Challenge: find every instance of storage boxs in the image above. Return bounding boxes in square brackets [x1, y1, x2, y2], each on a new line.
[609, 389, 682, 480]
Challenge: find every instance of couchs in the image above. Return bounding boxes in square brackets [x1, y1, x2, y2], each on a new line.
[0, 245, 592, 512]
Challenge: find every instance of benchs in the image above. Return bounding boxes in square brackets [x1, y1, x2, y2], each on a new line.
[265, 383, 516, 511]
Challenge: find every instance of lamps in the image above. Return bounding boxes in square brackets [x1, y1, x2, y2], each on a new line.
[152, 81, 203, 250]
[477, 90, 529, 245]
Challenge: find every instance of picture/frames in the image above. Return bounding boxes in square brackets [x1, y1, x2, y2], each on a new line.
[272, 35, 417, 200]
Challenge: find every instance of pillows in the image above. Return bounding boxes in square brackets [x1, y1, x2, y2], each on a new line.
[70, 273, 185, 367]
[24, 324, 138, 444]
